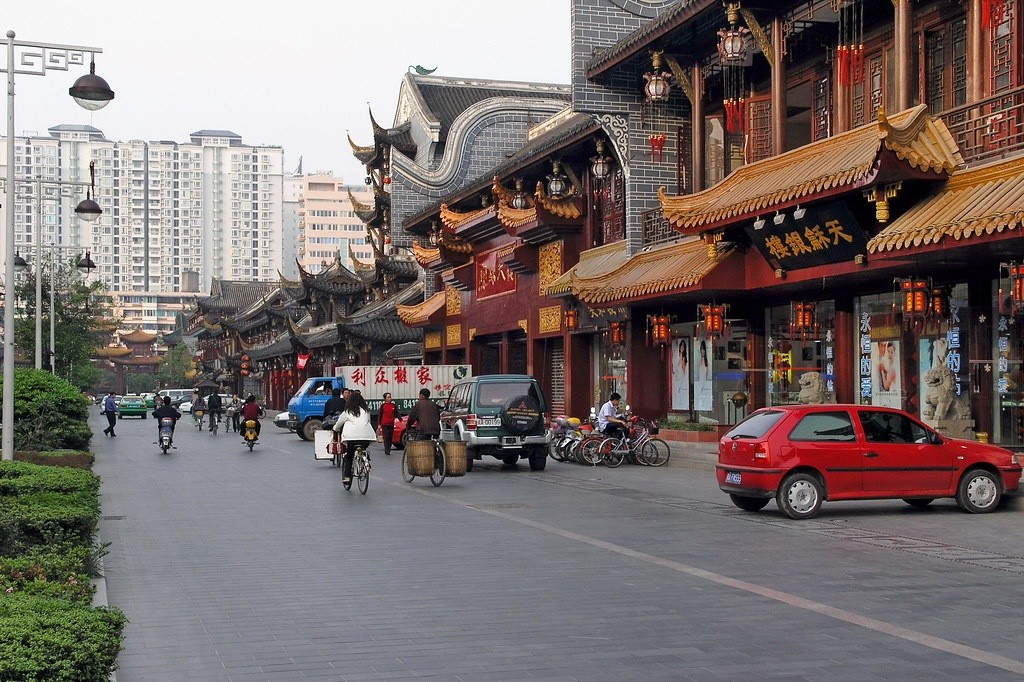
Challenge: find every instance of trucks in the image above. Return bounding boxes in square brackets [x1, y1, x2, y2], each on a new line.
[287, 365, 473, 441]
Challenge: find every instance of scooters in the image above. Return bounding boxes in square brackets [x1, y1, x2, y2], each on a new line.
[154, 400, 163, 412]
[153, 418, 178, 455]
[240, 413, 260, 452]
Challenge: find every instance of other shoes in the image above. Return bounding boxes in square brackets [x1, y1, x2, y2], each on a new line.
[104, 430, 108, 436]
[342, 477, 351, 482]
[385, 449, 390, 455]
[112, 433, 117, 436]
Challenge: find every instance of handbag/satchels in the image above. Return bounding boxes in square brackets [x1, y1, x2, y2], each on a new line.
[378, 431, 384, 443]
[328, 433, 346, 454]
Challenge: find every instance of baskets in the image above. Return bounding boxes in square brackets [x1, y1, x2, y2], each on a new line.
[226, 410, 235, 416]
[648, 424, 659, 434]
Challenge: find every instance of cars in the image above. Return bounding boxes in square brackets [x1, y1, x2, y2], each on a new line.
[714, 402, 1022, 520]
[273, 413, 297, 434]
[99, 395, 122, 416]
[116, 393, 148, 419]
[168, 394, 192, 411]
[94, 393, 106, 405]
[139, 392, 157, 408]
[178, 393, 247, 413]
[376, 414, 417, 449]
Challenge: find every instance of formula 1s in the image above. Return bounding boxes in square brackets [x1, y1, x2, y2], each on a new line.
[224, 406, 237, 433]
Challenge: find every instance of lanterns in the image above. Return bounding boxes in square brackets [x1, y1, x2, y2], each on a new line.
[645, 299, 731, 361]
[288, 370, 294, 378]
[274, 370, 279, 377]
[242, 355, 250, 361]
[280, 369, 287, 377]
[240, 369, 250, 376]
[562, 303, 626, 352]
[242, 362, 249, 368]
[274, 378, 294, 394]
[789, 256, 1024, 348]
[383, 0, 865, 253]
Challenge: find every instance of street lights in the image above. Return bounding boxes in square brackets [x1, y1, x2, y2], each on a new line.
[0, 175, 104, 376]
[0, 27, 117, 468]
[13, 243, 98, 377]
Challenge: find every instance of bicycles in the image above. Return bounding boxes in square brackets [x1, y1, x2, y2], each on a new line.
[341, 444, 372, 495]
[547, 405, 671, 466]
[212, 408, 222, 436]
[327, 428, 348, 469]
[194, 411, 206, 432]
[258, 407, 268, 419]
[400, 427, 448, 488]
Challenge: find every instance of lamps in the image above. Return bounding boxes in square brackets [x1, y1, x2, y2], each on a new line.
[429, 222, 439, 245]
[365, 176, 371, 185]
[546, 160, 567, 198]
[717, 3, 749, 64]
[512, 179, 527, 210]
[643, 52, 671, 102]
[590, 139, 611, 180]
[753, 209, 806, 229]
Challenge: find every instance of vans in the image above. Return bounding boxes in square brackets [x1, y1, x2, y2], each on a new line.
[156, 388, 195, 405]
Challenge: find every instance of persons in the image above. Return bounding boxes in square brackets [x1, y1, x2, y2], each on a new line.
[323, 383, 332, 395]
[154, 393, 163, 410]
[672, 340, 689, 409]
[103, 392, 119, 437]
[208, 389, 239, 433]
[696, 340, 708, 381]
[191, 392, 199, 418]
[192, 394, 207, 419]
[321, 387, 441, 483]
[143, 394, 147, 402]
[240, 396, 263, 437]
[598, 392, 630, 440]
[874, 341, 896, 391]
[242, 392, 253, 405]
[152, 396, 181, 443]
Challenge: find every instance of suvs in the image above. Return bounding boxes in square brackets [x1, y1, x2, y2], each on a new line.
[439, 374, 553, 470]
